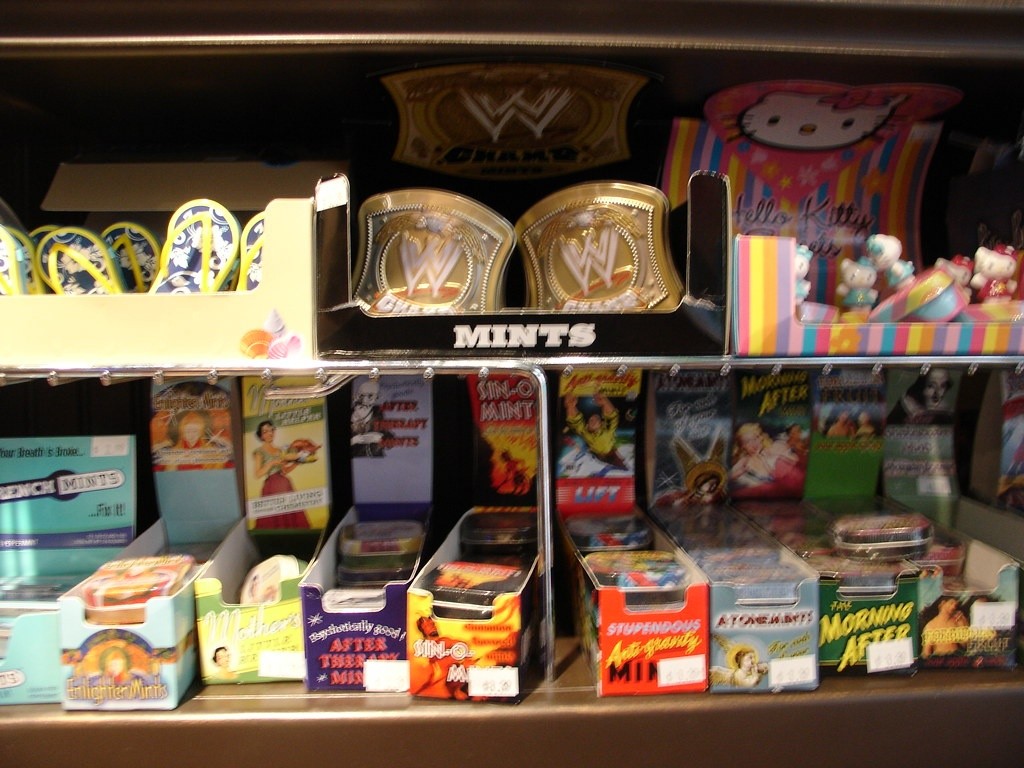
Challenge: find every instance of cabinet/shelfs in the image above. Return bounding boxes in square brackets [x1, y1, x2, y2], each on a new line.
[0, 0, 1024, 768]
